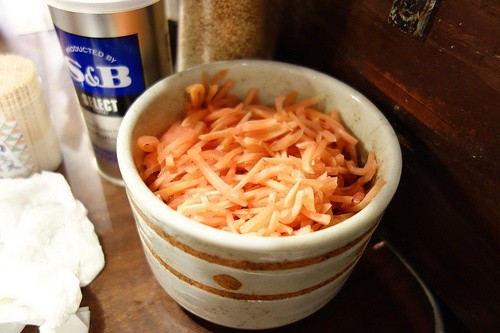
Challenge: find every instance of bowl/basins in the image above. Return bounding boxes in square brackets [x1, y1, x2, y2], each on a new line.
[116, 58, 404, 331]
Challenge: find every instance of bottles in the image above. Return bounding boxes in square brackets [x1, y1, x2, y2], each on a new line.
[48, 1, 174, 187]
